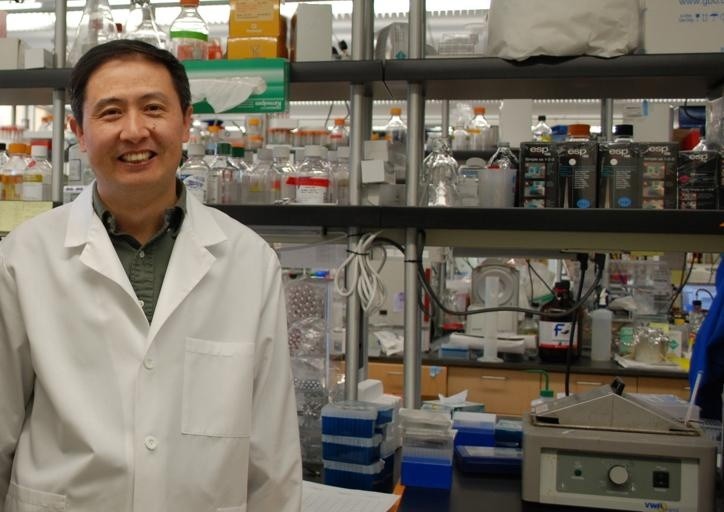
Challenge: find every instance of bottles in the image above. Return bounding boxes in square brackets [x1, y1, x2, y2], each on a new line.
[0, 102, 640, 209]
[65, 0, 210, 63]
[535, 275, 580, 365]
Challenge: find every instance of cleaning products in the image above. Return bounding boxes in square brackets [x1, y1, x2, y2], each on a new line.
[589, 288, 615, 361]
[521, 313, 539, 358]
[537, 280, 582, 359]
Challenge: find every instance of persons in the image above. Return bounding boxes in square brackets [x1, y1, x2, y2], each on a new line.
[0, 38, 304, 512]
